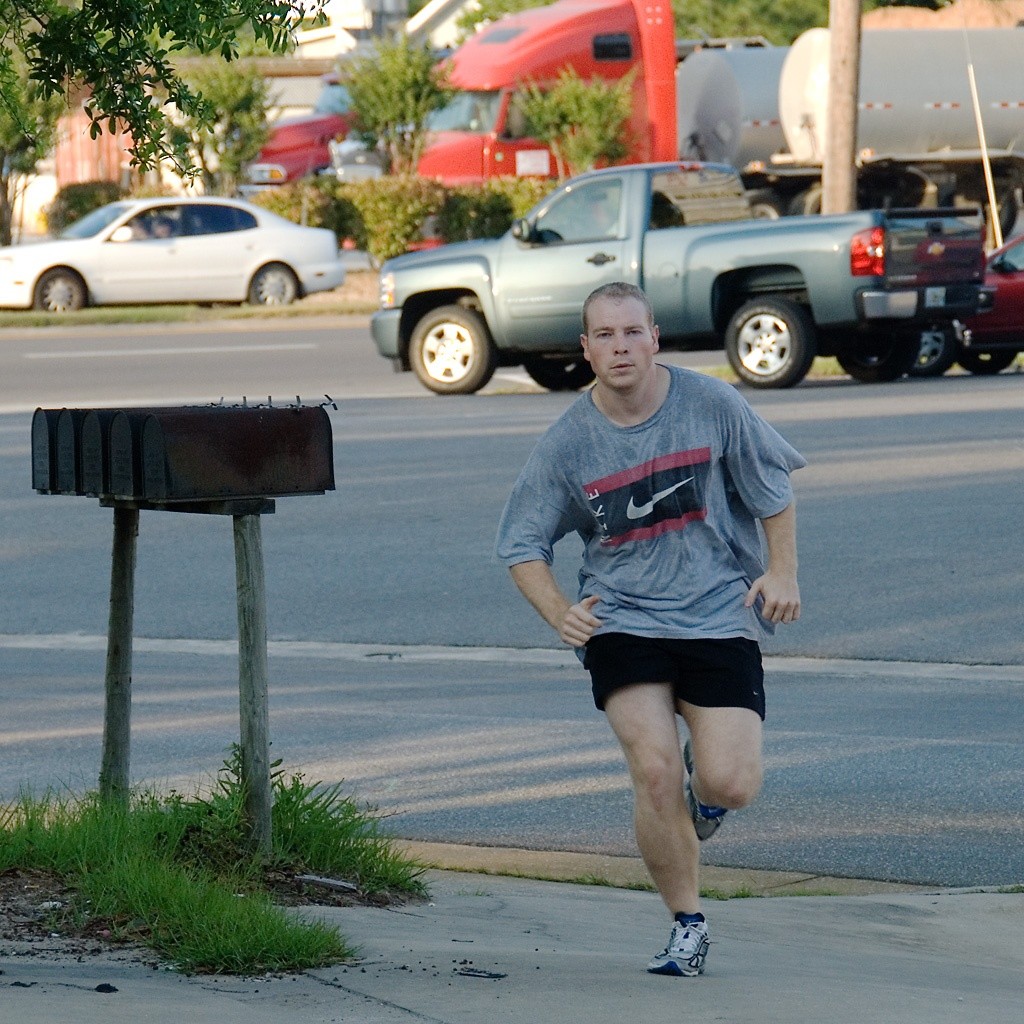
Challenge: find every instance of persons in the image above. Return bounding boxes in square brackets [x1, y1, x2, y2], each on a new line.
[495, 281, 806, 979]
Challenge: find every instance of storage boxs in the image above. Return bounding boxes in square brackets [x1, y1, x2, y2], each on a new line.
[29, 408, 336, 501]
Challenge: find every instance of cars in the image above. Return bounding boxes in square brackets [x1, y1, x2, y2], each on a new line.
[905, 232, 1024, 381]
[0, 193, 346, 314]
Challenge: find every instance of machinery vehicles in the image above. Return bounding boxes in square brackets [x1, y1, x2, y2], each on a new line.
[410, 1, 1024, 240]
[239, 33, 789, 193]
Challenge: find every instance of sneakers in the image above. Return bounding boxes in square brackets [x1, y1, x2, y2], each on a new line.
[646, 915, 710, 976]
[683, 739, 728, 840]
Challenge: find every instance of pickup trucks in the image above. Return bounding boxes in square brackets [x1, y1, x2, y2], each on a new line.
[368, 158, 998, 397]
